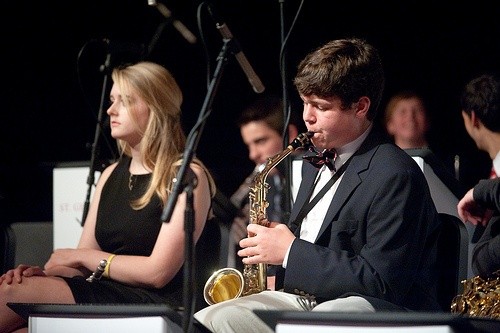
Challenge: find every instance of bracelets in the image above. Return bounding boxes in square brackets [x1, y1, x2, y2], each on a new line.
[106, 254, 116, 279]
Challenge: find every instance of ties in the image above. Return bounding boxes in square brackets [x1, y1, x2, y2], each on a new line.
[490, 168, 496, 181]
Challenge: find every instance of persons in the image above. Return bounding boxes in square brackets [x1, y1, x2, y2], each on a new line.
[0, 62, 221, 333]
[208, 37, 500, 333]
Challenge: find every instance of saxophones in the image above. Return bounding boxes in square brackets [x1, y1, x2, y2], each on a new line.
[203, 130, 315, 306]
[450, 270, 500, 316]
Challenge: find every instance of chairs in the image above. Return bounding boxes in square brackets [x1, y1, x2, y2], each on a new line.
[405, 213, 469, 310]
[196, 217, 236, 309]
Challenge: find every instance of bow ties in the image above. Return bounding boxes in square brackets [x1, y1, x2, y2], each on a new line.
[302, 146, 337, 174]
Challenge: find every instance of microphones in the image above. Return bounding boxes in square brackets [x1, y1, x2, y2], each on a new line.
[147, 0, 197, 43]
[100, 38, 127, 56]
[207, 2, 266, 94]
